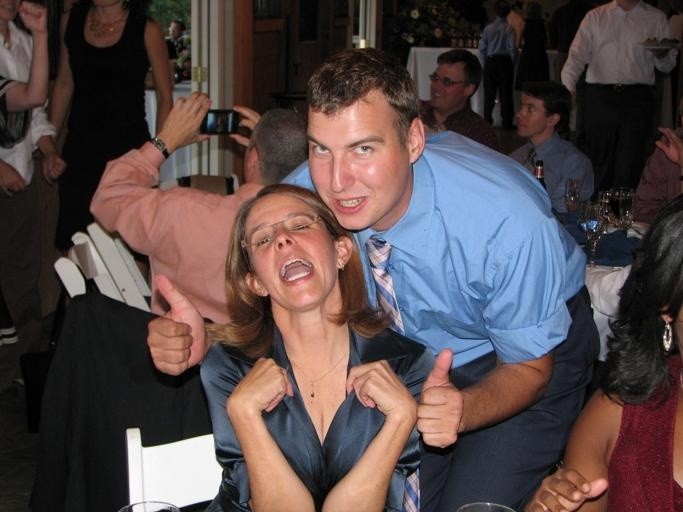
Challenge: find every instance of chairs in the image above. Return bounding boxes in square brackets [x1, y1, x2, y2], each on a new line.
[51, 229, 129, 307]
[176, 174, 235, 199]
[84, 220, 151, 314]
[125, 427, 225, 512]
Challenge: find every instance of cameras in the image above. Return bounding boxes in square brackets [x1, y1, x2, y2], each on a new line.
[200, 108, 241, 135]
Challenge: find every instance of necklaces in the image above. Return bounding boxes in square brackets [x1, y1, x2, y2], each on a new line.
[286, 347, 350, 397]
[85, 5, 127, 39]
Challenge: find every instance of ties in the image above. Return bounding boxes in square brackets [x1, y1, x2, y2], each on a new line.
[523, 148, 536, 170]
[365, 238, 405, 336]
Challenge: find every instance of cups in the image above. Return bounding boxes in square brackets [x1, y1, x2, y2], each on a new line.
[565, 177, 580, 202]
[585, 207, 597, 235]
[594, 219, 603, 233]
[599, 190, 611, 215]
[619, 188, 629, 218]
[611, 188, 619, 217]
[578, 220, 586, 236]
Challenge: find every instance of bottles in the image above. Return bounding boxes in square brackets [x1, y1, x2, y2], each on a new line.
[451, 30, 478, 48]
[534, 160, 546, 191]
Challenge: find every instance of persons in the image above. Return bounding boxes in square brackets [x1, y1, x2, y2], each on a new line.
[522, 192, 683, 508]
[417, 49, 499, 152]
[278, 48, 600, 508]
[0, 0, 66, 408]
[90, 92, 309, 326]
[0, 3, 49, 344]
[632, 97, 683, 223]
[560, 0, 678, 202]
[509, 81, 595, 213]
[478, 1, 520, 130]
[199, 183, 455, 508]
[423, 25, 451, 47]
[164, 21, 186, 60]
[49, 0, 173, 251]
[514, 1, 550, 90]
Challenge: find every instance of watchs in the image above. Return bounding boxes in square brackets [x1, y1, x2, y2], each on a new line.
[148, 137, 171, 158]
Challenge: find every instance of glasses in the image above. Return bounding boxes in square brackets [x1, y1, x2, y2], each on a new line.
[239, 210, 324, 268]
[428, 73, 469, 87]
